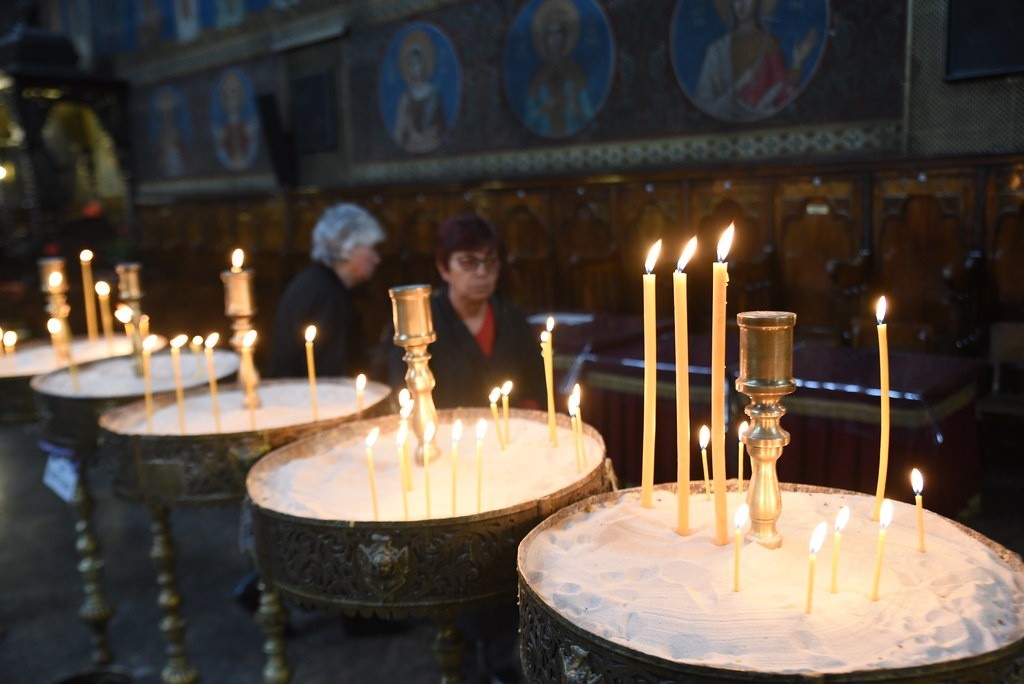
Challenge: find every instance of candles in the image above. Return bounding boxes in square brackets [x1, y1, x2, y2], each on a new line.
[642, 240, 662, 510]
[870, 497, 892, 601]
[738, 420, 749, 504]
[489, 380, 513, 450]
[873, 295, 890, 522]
[805, 522, 827, 613]
[831, 507, 850, 593]
[673, 235, 698, 535]
[734, 505, 749, 592]
[712, 220, 736, 546]
[540, 317, 557, 449]
[908, 467, 925, 554]
[0, 249, 490, 523]
[699, 425, 711, 501]
[567, 383, 586, 470]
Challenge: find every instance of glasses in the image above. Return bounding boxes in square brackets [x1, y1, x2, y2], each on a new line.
[457, 255, 498, 271]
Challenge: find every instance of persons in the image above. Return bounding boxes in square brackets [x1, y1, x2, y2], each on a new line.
[383, 214, 567, 414]
[254, 201, 410, 640]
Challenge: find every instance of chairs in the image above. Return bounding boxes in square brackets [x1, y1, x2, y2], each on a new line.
[983, 322, 1024, 473]
[850, 320, 934, 353]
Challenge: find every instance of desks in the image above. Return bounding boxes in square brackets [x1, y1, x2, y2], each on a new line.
[531, 312, 990, 523]
